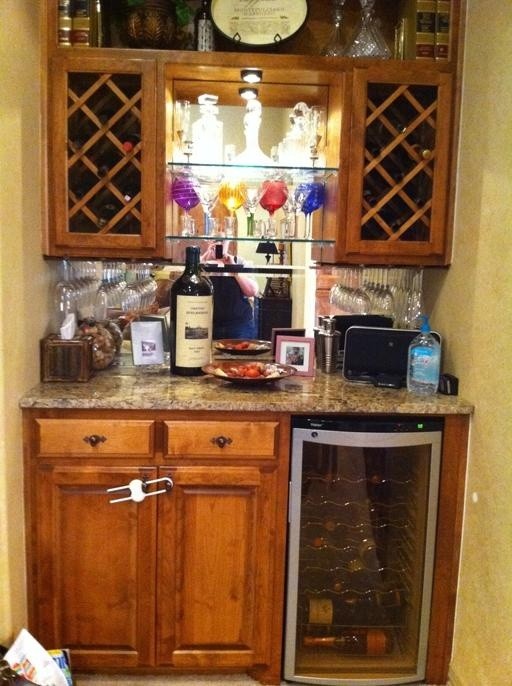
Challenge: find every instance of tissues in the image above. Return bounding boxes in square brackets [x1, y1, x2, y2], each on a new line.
[40, 313, 93, 382]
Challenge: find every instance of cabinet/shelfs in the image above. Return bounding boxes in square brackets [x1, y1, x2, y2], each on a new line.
[42, 0, 466, 269]
[21, 410, 291, 686]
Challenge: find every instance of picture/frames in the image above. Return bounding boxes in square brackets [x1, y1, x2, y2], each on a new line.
[271, 328, 305, 355]
[276, 335, 314, 376]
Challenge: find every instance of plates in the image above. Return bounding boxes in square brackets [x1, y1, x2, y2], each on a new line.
[201, 359, 297, 385]
[211, 339, 272, 354]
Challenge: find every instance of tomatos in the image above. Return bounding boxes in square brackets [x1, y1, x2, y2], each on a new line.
[240, 364, 260, 377]
[225, 341, 249, 351]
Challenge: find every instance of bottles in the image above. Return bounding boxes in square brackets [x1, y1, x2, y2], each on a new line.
[304, 469, 404, 656]
[97, 131, 141, 235]
[195, 0, 216, 51]
[321, 1, 392, 60]
[317, 318, 341, 374]
[191, 94, 309, 168]
[170, 246, 212, 376]
[364, 122, 431, 201]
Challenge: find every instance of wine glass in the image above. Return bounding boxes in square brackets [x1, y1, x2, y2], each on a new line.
[308, 107, 324, 167]
[175, 100, 188, 146]
[172, 174, 326, 241]
[53, 260, 158, 323]
[330, 267, 423, 329]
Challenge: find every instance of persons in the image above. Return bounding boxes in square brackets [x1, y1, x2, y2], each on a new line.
[287, 347, 303, 365]
[195, 225, 258, 340]
